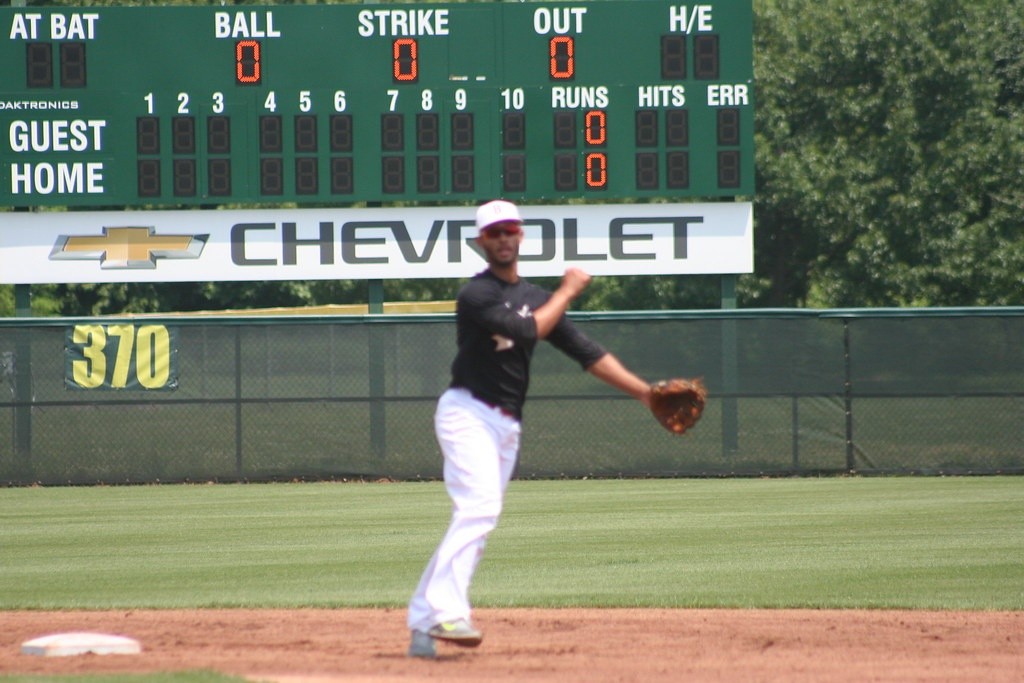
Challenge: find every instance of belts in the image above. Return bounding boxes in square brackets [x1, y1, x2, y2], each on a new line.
[458, 387, 512, 418]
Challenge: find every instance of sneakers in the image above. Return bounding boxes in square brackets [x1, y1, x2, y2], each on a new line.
[408, 628, 437, 657]
[428, 617, 483, 645]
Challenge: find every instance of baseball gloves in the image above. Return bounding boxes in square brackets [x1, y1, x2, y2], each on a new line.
[648, 378, 706, 435]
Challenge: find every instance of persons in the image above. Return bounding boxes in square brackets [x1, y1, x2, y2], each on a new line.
[408, 199, 704, 659]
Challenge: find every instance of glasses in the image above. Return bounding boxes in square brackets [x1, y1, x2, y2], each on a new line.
[485, 224, 520, 239]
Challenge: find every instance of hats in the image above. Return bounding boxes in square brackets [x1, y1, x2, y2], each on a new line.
[476, 199, 523, 231]
